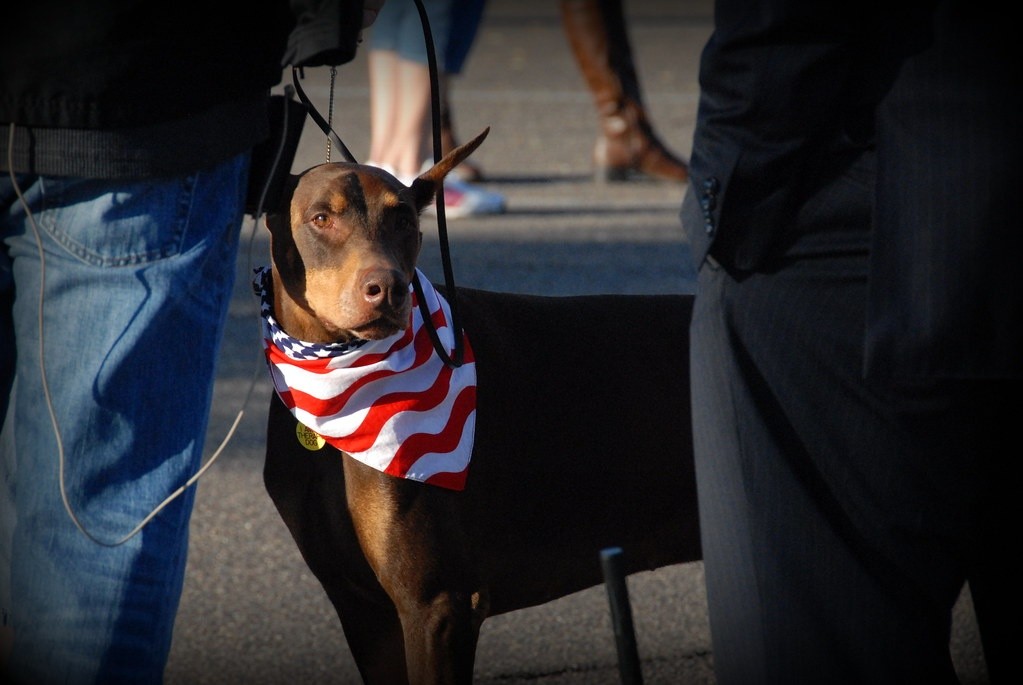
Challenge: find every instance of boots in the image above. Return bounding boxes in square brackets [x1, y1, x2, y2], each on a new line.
[559, 0, 690, 183]
[430, 76, 481, 182]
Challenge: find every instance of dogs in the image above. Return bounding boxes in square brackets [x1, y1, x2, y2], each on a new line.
[244, 127, 702, 685]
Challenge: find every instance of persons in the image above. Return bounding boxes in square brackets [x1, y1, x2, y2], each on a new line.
[369, 1, 507, 222]
[681, 0, 1023, 685]
[560, 0, 689, 184]
[0, 0, 382, 685]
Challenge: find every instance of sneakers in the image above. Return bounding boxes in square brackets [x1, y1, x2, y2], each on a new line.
[362, 157, 507, 218]
